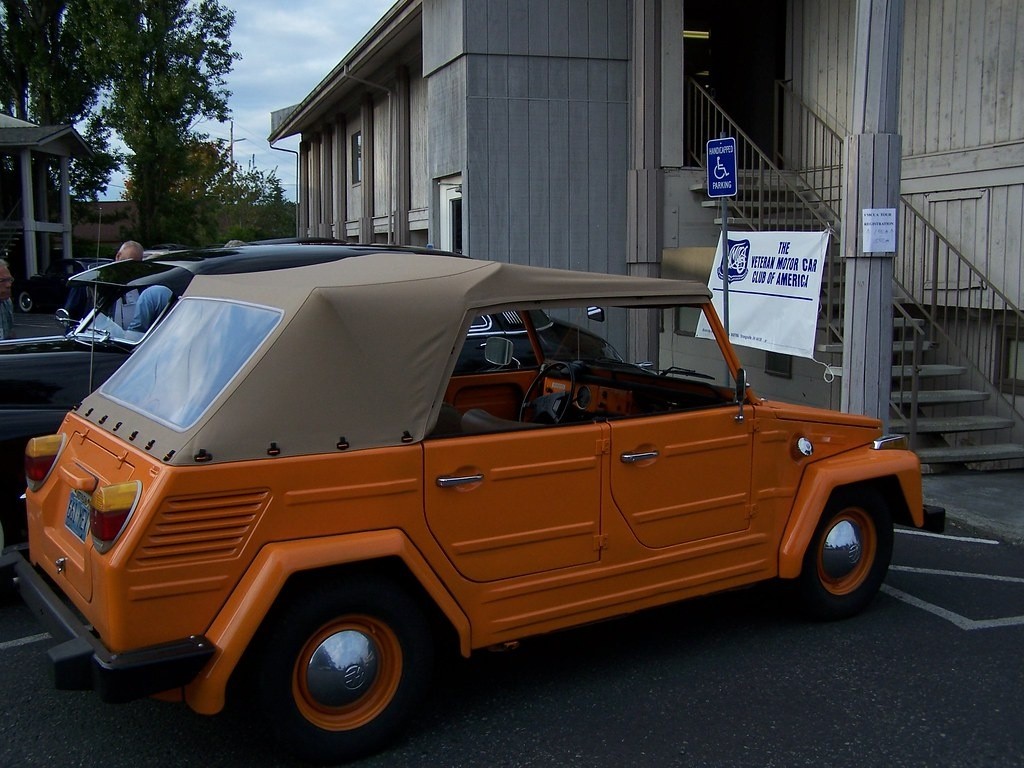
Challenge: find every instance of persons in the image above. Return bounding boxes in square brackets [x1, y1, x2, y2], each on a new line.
[225, 240, 246, 248]
[64, 241, 147, 337]
[0, 259, 17, 340]
[126, 285, 174, 340]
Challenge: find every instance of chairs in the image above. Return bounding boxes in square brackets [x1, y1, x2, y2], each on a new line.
[460, 408, 543, 435]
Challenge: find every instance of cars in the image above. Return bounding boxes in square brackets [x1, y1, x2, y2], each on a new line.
[0, 236, 657, 544]
[0, 252, 946, 764]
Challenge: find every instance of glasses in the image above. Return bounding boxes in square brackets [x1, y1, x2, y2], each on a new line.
[0, 277, 14, 283]
